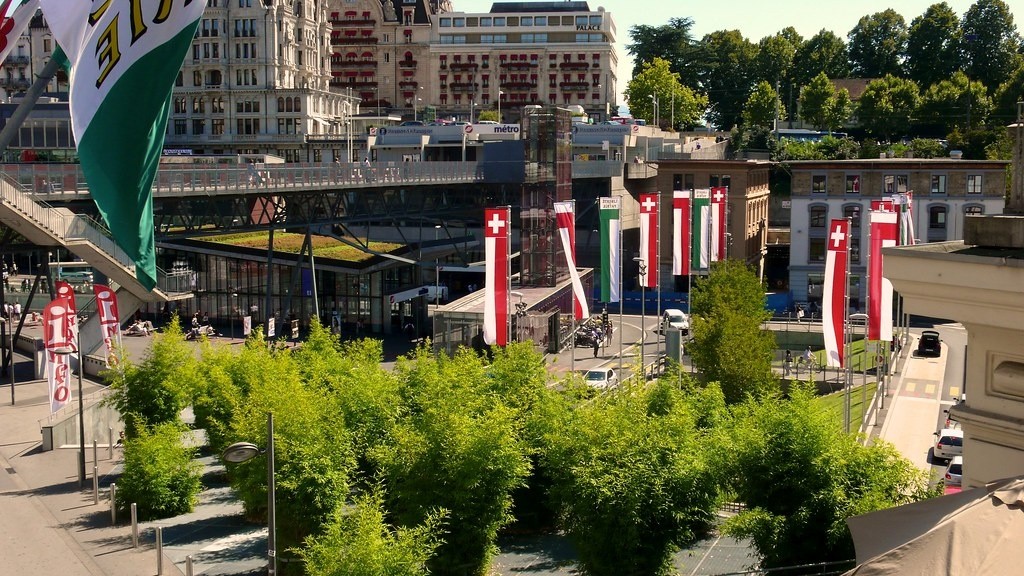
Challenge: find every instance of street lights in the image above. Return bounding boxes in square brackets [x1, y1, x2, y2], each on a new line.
[414, 86, 424, 121]
[435, 225, 442, 310]
[47, 252, 53, 274]
[230, 293, 238, 340]
[647, 90, 656, 128]
[498, 87, 504, 123]
[632, 257, 648, 375]
[371, 87, 380, 116]
[470, 99, 478, 124]
[652, 98, 660, 128]
[222, 412, 276, 576]
[507, 291, 524, 344]
[53, 332, 87, 491]
[0, 307, 15, 406]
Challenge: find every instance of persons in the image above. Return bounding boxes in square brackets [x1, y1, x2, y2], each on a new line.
[363, 156, 371, 181]
[403, 157, 411, 181]
[593, 336, 601, 358]
[796, 306, 802, 324]
[22, 278, 27, 291]
[803, 346, 817, 370]
[333, 157, 340, 184]
[4, 301, 21, 320]
[32, 312, 38, 321]
[11, 285, 18, 293]
[41, 279, 46, 293]
[248, 158, 265, 184]
[605, 324, 613, 347]
[28, 278, 31, 291]
[11, 262, 18, 276]
[5, 277, 10, 290]
[126, 319, 151, 337]
[185, 309, 223, 341]
[785, 354, 792, 375]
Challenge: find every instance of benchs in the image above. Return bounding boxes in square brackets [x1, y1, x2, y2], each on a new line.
[14, 313, 215, 341]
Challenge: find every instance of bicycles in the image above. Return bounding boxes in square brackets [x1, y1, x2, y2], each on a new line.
[795, 358, 822, 374]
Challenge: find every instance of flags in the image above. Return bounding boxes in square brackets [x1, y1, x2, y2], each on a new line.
[93, 284, 122, 373]
[482, 185, 916, 367]
[54, 280, 78, 359]
[43, 296, 72, 413]
[1, 0, 210, 292]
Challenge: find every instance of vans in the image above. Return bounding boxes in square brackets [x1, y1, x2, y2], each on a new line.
[662, 309, 688, 336]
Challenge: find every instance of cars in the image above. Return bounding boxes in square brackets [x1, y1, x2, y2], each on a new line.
[944, 455, 963, 487]
[397, 111, 647, 132]
[933, 393, 966, 462]
[918, 331, 944, 357]
[63, 272, 93, 284]
[582, 367, 619, 393]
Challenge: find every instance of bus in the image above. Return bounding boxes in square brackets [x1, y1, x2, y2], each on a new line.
[768, 128, 847, 153]
[48, 261, 93, 284]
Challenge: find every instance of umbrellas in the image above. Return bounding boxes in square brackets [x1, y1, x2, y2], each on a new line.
[841, 474, 1024, 576]
